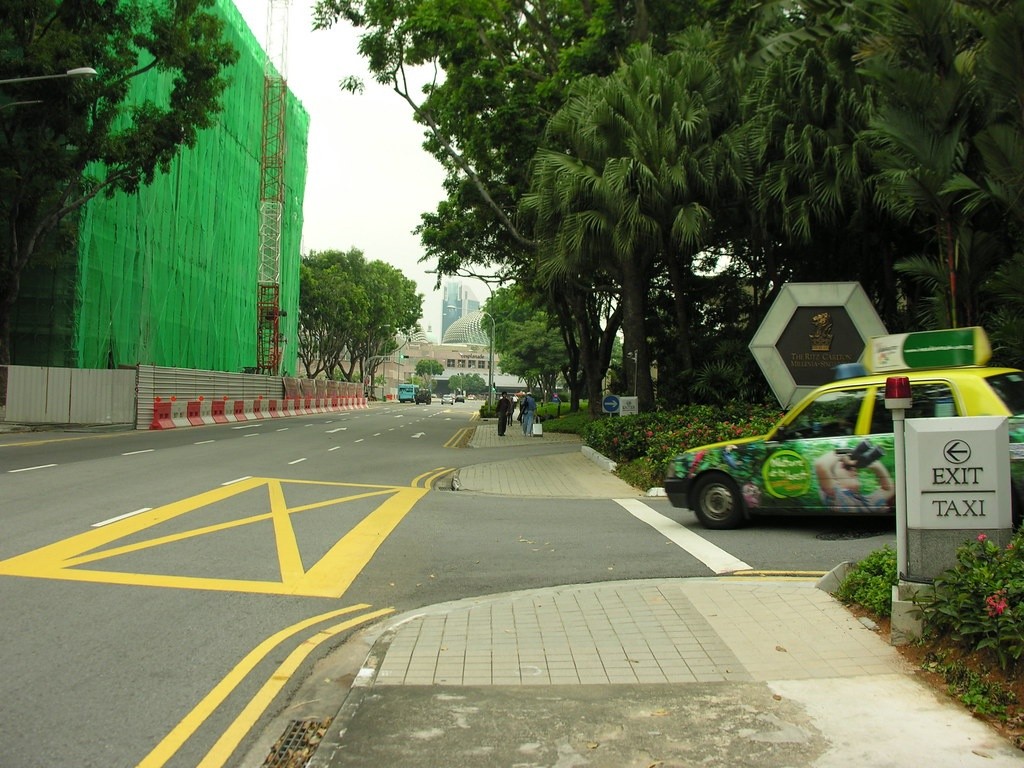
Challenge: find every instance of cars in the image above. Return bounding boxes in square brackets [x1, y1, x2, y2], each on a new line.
[441, 395, 453, 405]
[662, 326, 1024, 530]
[432, 394, 437, 398]
[455, 395, 464, 403]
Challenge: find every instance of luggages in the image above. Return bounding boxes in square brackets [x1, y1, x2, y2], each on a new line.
[533, 416, 543, 438]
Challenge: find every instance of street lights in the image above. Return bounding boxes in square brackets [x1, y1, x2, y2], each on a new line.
[445, 306, 495, 414]
[366, 324, 390, 400]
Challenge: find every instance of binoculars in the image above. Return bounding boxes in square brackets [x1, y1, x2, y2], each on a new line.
[844, 439, 887, 471]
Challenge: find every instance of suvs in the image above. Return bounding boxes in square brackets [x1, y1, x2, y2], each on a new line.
[467, 395, 474, 400]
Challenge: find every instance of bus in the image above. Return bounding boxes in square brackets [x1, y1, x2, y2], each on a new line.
[397, 384, 419, 403]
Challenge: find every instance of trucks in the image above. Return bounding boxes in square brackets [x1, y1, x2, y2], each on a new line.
[415, 388, 432, 405]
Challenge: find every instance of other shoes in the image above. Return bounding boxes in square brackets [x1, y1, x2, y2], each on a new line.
[522, 432, 526, 437]
[527, 434, 531, 437]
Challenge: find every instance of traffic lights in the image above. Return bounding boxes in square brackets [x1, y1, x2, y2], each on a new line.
[493, 382, 496, 389]
[400, 355, 409, 358]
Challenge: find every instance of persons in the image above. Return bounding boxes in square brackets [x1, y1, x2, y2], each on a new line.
[496, 391, 519, 436]
[816, 452, 894, 512]
[520, 392, 538, 437]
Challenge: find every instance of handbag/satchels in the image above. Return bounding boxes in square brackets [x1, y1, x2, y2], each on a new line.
[517, 413, 522, 421]
